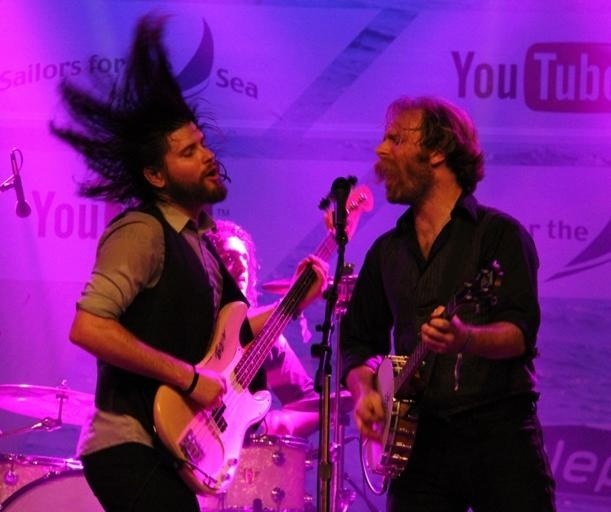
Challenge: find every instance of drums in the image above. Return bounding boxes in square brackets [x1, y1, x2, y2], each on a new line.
[1, 449, 106, 512]
[201, 432, 308, 511]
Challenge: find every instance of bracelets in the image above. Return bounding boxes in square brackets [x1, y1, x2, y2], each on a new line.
[184, 372, 200, 396]
[459, 328, 472, 353]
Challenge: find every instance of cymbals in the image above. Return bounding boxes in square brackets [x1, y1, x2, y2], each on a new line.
[284, 388, 354, 415]
[257, 278, 349, 296]
[0, 382, 97, 428]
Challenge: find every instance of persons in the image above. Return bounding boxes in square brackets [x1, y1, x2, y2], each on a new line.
[334, 92, 560, 511]
[47, 7, 330, 512]
[200, 218, 321, 440]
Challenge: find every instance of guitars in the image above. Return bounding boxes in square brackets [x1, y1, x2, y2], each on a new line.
[152, 183, 375, 497]
[361, 259, 503, 482]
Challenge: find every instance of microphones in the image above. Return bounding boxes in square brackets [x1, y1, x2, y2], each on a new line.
[330, 172, 353, 243]
[7, 147, 31, 218]
